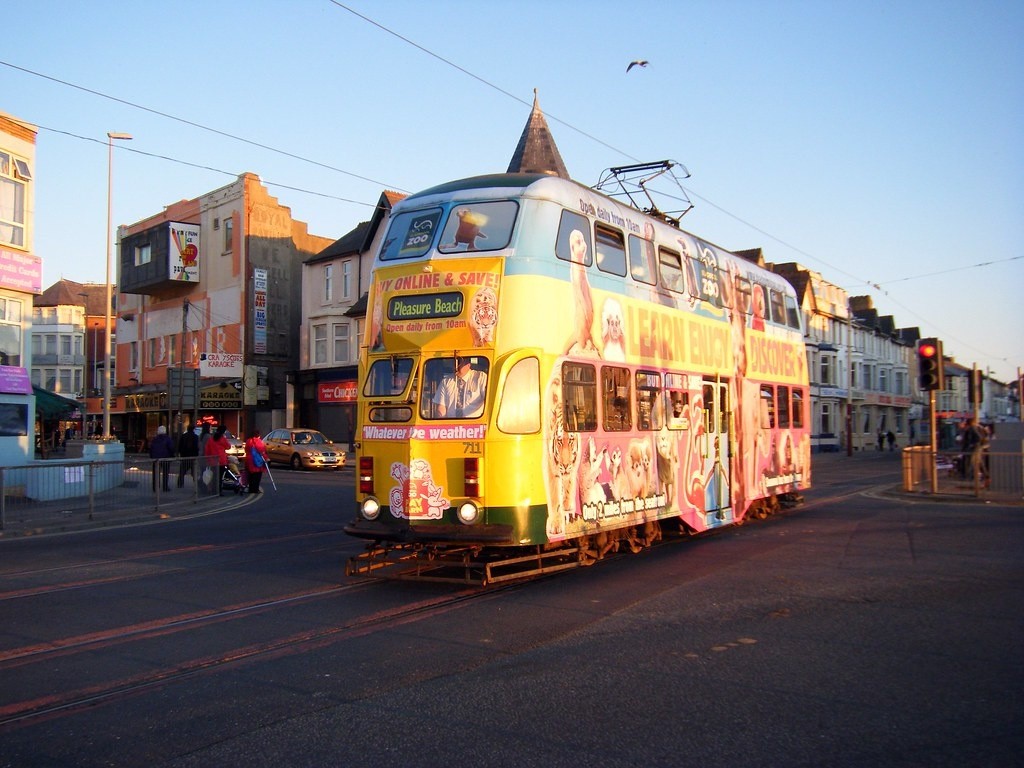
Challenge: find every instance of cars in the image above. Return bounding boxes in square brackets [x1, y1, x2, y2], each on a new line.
[97, 419, 126, 435]
[260, 428, 347, 470]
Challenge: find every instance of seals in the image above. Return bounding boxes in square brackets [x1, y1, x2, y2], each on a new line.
[439, 207, 488, 251]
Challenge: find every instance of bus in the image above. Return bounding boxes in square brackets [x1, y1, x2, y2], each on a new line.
[342, 169, 813, 585]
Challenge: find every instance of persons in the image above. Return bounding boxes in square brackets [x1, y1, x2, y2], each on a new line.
[95, 422, 103, 440]
[431, 356, 487, 418]
[206, 424, 231, 496]
[956, 420, 996, 487]
[245, 429, 270, 493]
[228, 455, 242, 487]
[176, 423, 199, 488]
[65, 425, 75, 440]
[149, 425, 175, 493]
[302, 433, 312, 444]
[876, 429, 897, 451]
[197, 422, 210, 475]
[55, 428, 60, 446]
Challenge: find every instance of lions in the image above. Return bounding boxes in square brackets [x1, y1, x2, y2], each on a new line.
[649, 391, 680, 508]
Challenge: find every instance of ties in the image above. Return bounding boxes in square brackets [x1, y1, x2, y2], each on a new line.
[456, 379, 465, 417]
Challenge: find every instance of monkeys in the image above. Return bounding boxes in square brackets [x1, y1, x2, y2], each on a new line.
[725, 262, 752, 376]
[602, 314, 627, 363]
[602, 448, 632, 501]
[562, 229, 602, 359]
[625, 437, 652, 500]
[577, 437, 607, 512]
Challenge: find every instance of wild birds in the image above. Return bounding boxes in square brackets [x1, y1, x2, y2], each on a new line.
[626, 60, 649, 72]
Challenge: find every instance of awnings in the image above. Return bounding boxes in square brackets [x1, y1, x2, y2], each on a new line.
[33, 383, 81, 419]
[112, 384, 168, 394]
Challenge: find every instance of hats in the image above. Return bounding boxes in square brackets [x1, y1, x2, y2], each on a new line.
[228, 455, 238, 463]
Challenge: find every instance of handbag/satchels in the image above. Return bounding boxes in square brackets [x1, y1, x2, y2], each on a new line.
[203, 466, 213, 485]
[252, 448, 264, 467]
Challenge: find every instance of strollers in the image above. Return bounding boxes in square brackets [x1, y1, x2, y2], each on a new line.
[220, 465, 248, 496]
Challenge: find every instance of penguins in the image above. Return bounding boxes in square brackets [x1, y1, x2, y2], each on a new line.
[704, 436, 730, 520]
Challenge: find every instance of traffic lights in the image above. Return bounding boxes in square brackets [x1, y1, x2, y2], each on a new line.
[917, 337, 946, 391]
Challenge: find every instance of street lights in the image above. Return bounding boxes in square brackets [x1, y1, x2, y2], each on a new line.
[99, 128, 134, 441]
